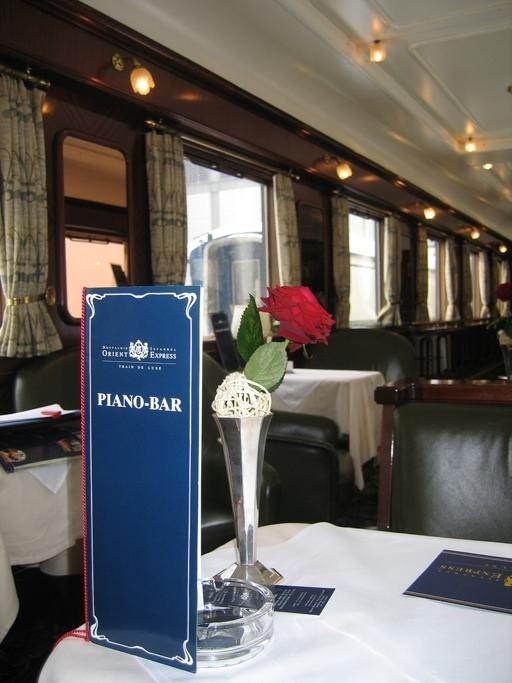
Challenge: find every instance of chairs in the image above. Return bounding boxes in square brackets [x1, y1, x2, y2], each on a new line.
[371, 363, 510, 548]
[303, 326, 423, 399]
[198, 361, 359, 535]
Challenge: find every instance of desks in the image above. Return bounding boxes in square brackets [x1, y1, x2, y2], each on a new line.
[34, 514, 511, 683]
[262, 361, 387, 494]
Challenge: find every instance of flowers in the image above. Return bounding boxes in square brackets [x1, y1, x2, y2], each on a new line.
[232, 283, 335, 391]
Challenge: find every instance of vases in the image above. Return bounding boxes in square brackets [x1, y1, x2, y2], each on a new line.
[208, 405, 284, 593]
